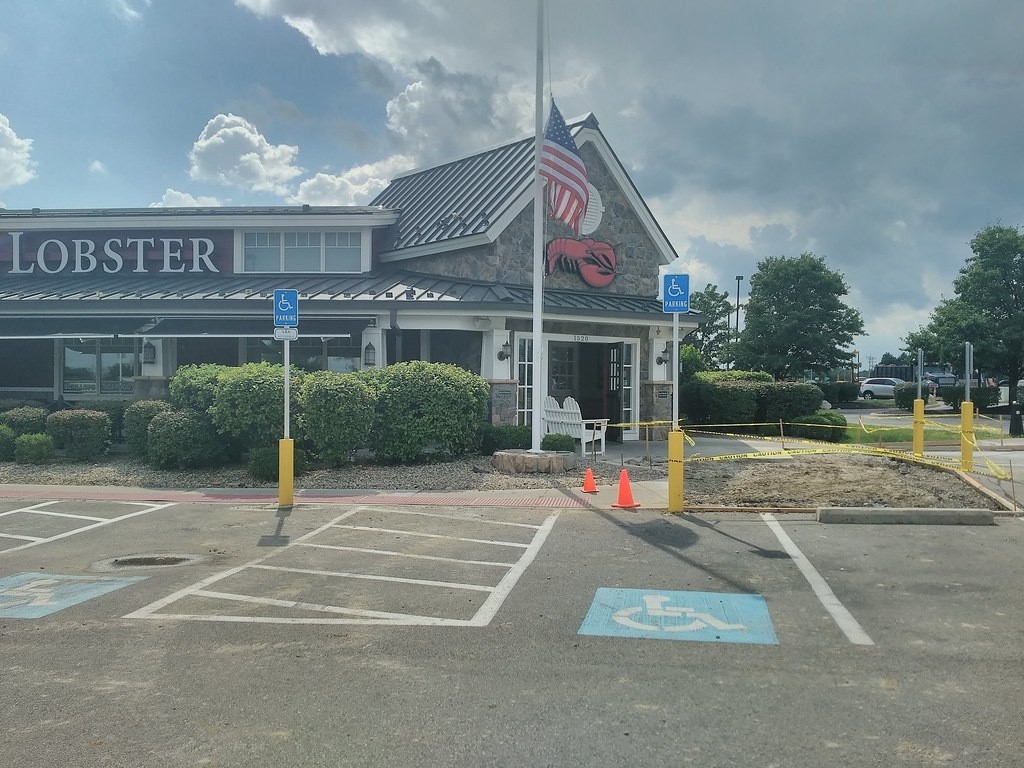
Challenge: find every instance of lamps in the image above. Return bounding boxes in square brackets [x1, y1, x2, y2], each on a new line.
[143, 341, 156, 364]
[496, 340, 512, 361]
[364, 341, 376, 365]
[656, 347, 670, 365]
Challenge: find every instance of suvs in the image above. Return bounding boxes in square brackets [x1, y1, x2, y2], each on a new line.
[860, 378, 907, 400]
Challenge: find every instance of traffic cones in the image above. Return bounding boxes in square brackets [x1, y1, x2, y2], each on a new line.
[581, 468, 599, 493]
[611, 469, 641, 508]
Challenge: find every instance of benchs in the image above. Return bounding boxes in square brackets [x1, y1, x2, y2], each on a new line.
[543, 396, 611, 458]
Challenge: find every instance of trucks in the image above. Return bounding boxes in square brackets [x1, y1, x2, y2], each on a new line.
[874, 364, 959, 394]
